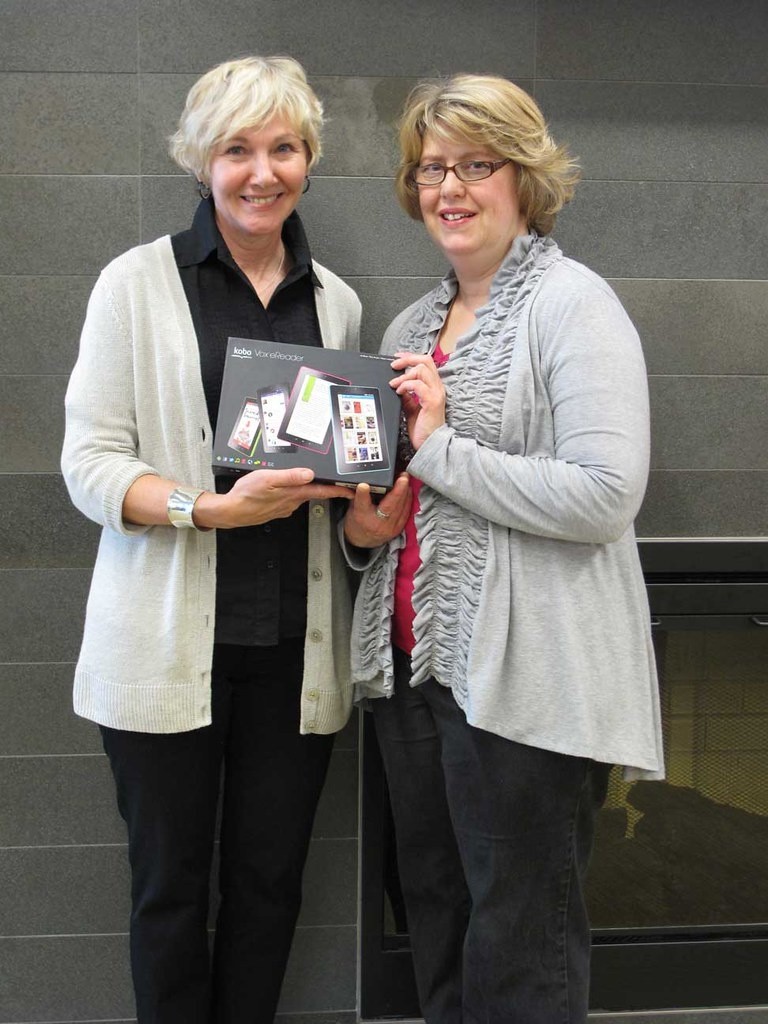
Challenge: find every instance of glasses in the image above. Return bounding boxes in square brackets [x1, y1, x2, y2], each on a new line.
[409, 157, 512, 186]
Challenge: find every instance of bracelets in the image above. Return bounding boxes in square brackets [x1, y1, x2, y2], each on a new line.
[168, 487, 214, 532]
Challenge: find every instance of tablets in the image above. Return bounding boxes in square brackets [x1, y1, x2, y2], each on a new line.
[226, 367, 391, 475]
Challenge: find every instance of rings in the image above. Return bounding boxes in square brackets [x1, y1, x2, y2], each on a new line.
[376, 506, 391, 519]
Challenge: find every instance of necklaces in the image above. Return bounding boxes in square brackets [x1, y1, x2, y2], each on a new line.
[258, 247, 285, 295]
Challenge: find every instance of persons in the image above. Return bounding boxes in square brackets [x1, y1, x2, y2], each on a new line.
[330, 68, 672, 1023]
[57, 54, 365, 1023]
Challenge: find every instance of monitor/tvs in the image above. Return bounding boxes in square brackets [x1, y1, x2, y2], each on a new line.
[380, 581, 767, 947]
